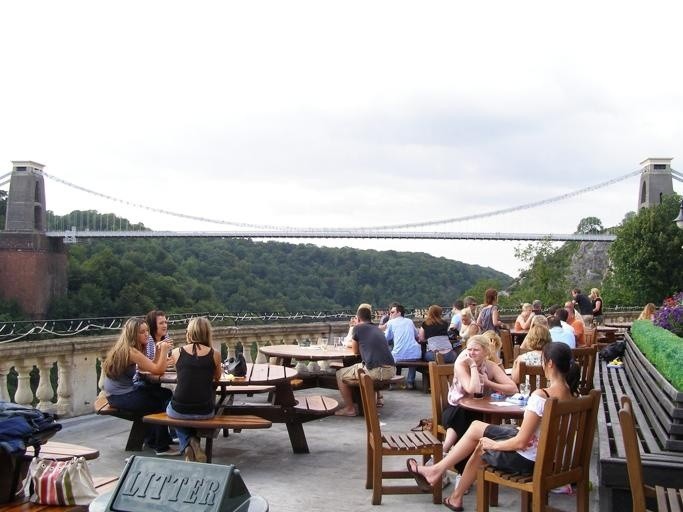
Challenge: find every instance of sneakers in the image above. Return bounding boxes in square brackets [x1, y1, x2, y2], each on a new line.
[425, 458, 449, 488]
[455, 474, 469, 494]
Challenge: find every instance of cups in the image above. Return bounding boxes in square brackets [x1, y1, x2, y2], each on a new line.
[473, 375, 483, 400]
[298, 336, 347, 352]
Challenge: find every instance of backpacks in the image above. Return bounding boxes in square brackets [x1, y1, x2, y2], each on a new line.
[600, 340, 626, 360]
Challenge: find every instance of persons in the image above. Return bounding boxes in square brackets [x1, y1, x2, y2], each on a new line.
[134, 309, 180, 443]
[165, 317, 222, 464]
[636, 302, 656, 322]
[102, 317, 183, 457]
[423, 334, 519, 496]
[333, 287, 603, 417]
[406, 342, 582, 512]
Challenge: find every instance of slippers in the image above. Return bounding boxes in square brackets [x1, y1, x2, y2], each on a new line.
[407, 458, 433, 492]
[443, 496, 464, 511]
[607, 356, 624, 368]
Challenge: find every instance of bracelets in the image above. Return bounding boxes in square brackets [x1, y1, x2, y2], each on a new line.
[469, 364, 477, 369]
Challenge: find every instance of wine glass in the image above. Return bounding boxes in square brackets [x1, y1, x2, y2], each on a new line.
[520, 384, 530, 409]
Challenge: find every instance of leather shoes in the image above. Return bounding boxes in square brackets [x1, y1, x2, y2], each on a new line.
[154, 437, 207, 463]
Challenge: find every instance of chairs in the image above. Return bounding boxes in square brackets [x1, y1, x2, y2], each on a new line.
[476, 387, 602, 511]
[429, 360, 455, 450]
[571, 344, 597, 395]
[619, 395, 683, 512]
[585, 327, 597, 347]
[501, 330, 511, 369]
[358, 368, 442, 505]
[517, 361, 547, 427]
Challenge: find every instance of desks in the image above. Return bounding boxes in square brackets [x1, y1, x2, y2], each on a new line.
[138, 360, 309, 463]
[260, 345, 362, 416]
[510, 328, 529, 333]
[605, 323, 632, 331]
[458, 396, 527, 507]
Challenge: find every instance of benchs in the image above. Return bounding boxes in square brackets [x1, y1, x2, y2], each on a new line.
[397, 360, 429, 391]
[330, 362, 344, 369]
[217, 385, 276, 402]
[596, 332, 683, 512]
[25, 441, 100, 461]
[0, 477, 119, 512]
[293, 395, 339, 423]
[217, 386, 277, 437]
[597, 326, 618, 343]
[143, 412, 272, 463]
[383, 374, 405, 383]
[95, 396, 150, 451]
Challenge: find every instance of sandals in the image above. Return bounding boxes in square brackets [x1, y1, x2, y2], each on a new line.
[335, 397, 384, 416]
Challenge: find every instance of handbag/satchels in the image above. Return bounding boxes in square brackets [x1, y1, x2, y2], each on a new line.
[224, 353, 247, 376]
[0, 401, 62, 456]
[15, 457, 100, 506]
[448, 328, 459, 340]
